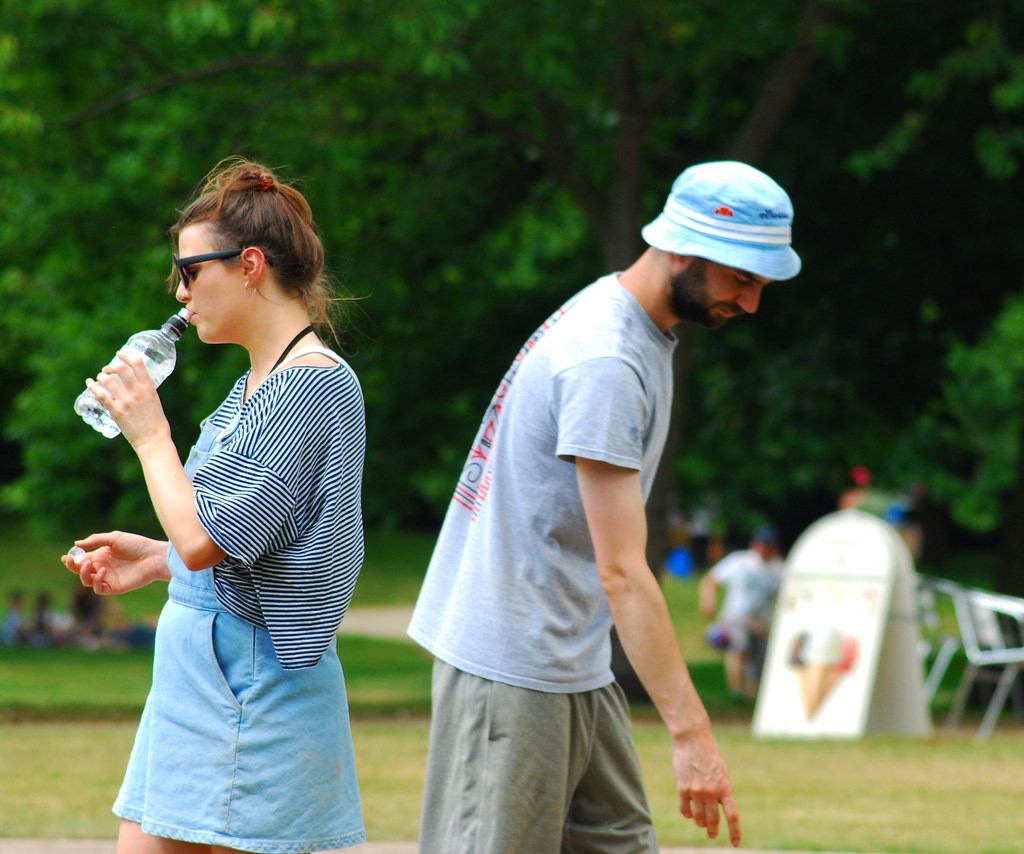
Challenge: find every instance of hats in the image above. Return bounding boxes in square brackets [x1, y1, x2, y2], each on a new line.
[757, 526, 778, 544]
[641, 161, 802, 282]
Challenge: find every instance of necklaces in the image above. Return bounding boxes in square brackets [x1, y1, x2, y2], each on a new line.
[258, 324, 314, 376]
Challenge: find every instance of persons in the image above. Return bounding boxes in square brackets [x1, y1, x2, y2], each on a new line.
[686, 465, 957, 702]
[1, 574, 132, 655]
[407, 161, 800, 854]
[61, 154, 366, 854]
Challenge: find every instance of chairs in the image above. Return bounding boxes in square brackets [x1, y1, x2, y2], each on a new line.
[921, 577, 1024, 743]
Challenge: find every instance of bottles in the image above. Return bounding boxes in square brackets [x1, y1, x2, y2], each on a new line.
[73, 307, 191, 438]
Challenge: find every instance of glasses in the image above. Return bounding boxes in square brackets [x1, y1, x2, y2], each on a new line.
[173, 247, 276, 288]
